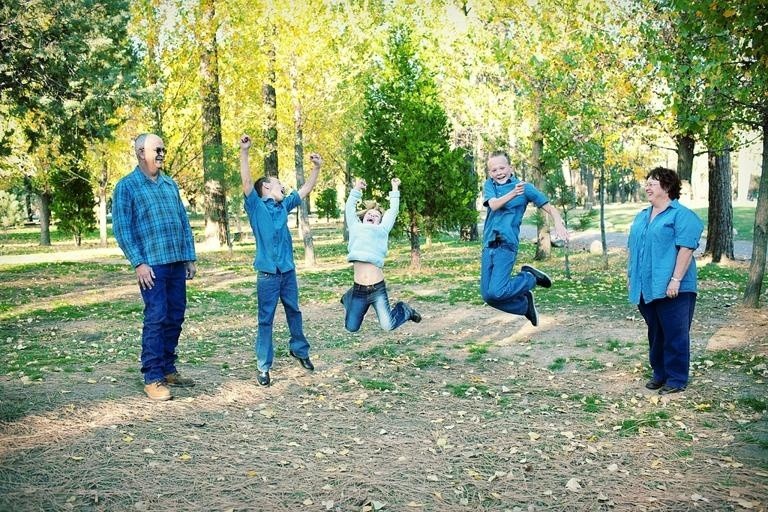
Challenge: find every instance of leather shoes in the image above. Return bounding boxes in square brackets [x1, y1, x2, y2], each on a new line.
[258, 370, 270, 387]
[289, 351, 314, 369]
[658, 384, 687, 395]
[645, 381, 662, 390]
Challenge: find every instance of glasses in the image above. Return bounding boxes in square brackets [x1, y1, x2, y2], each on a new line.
[145, 147, 165, 154]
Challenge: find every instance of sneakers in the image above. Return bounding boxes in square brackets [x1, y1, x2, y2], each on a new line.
[145, 381, 173, 400]
[522, 266, 553, 289]
[406, 304, 421, 323]
[164, 372, 194, 386]
[526, 290, 538, 326]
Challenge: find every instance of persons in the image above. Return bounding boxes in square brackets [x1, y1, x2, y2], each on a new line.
[112, 133, 197, 400]
[480, 150, 570, 327]
[340, 177, 422, 332]
[240, 135, 321, 385]
[626, 168, 704, 394]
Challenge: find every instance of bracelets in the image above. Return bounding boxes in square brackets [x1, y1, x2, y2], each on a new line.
[670, 277, 682, 282]
